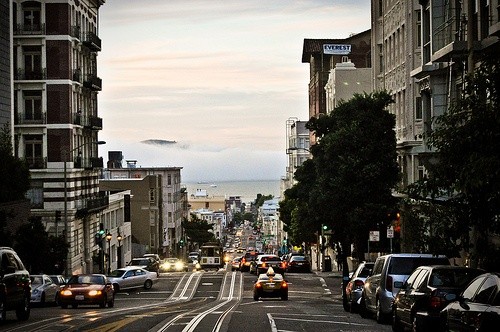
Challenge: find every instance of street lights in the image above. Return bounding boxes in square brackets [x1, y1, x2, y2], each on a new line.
[287, 147, 325, 272]
[106, 231, 112, 275]
[63, 140, 106, 278]
[116, 233, 123, 268]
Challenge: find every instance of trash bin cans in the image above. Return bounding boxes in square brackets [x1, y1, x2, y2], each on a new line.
[324, 255, 332, 272]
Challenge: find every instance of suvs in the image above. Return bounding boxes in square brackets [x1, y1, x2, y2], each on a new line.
[361, 254, 457, 324]
[0, 246, 31, 325]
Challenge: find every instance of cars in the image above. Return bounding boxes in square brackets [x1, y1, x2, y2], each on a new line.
[223, 223, 311, 278]
[343, 261, 376, 314]
[186, 252, 199, 263]
[48, 275, 66, 287]
[125, 253, 189, 276]
[391, 265, 487, 332]
[58, 274, 115, 309]
[106, 265, 157, 294]
[437, 272, 500, 332]
[28, 274, 57, 308]
[252, 267, 289, 302]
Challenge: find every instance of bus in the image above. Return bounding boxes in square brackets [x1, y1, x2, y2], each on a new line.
[197, 242, 222, 273]
